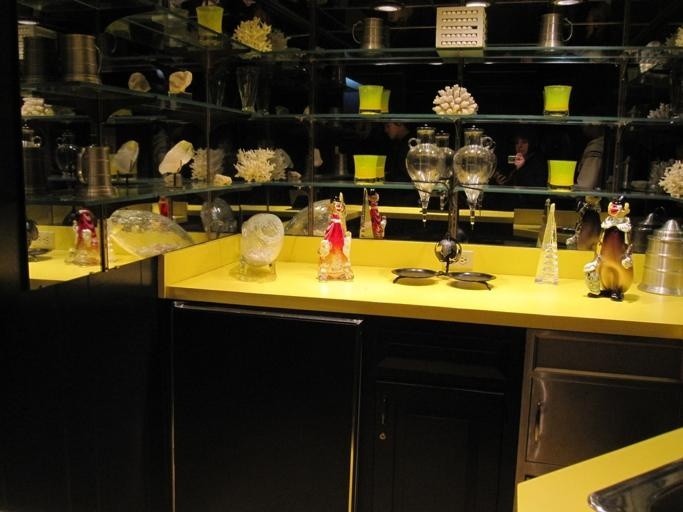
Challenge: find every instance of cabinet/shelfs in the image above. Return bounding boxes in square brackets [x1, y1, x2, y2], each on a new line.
[170, 303, 363, 512]
[359, 316, 525, 511]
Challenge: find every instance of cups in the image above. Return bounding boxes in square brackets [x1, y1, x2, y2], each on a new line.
[331, 154, 351, 179]
[195, 6, 224, 45]
[352, 155, 378, 185]
[60, 33, 104, 86]
[358, 85, 384, 114]
[377, 155, 387, 186]
[23, 35, 60, 85]
[164, 9, 190, 48]
[22, 147, 51, 196]
[543, 85, 573, 116]
[77, 147, 119, 199]
[537, 13, 575, 54]
[351, 17, 391, 56]
[547, 159, 577, 193]
[380, 89, 391, 114]
